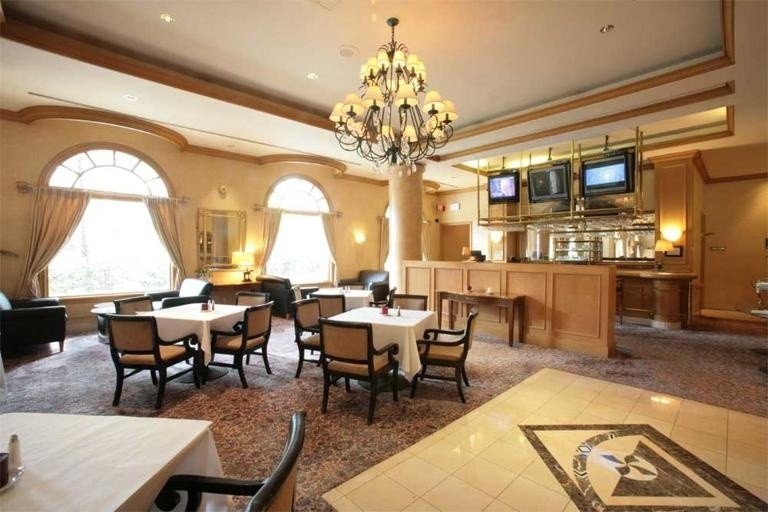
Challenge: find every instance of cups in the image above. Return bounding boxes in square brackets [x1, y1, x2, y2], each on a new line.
[488, 287, 494, 291]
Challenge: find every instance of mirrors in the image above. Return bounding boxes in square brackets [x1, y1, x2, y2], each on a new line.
[197, 207, 249, 272]
[516, 210, 657, 267]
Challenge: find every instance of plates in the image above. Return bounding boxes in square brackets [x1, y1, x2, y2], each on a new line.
[0, 467, 25, 492]
[486, 291, 496, 294]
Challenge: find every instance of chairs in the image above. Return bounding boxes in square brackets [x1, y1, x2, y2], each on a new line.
[153, 410, 307, 511]
[0, 291, 68, 353]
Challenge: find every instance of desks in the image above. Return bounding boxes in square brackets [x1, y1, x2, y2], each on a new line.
[436, 287, 528, 348]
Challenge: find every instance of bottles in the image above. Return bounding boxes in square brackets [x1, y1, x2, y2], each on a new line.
[202, 299, 215, 310]
[382, 306, 388, 313]
[397, 305, 401, 316]
[8, 434, 22, 472]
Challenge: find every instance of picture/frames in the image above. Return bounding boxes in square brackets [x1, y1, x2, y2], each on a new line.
[663, 246, 682, 258]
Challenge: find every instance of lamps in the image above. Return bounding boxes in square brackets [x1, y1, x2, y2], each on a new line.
[231, 251, 254, 282]
[328, 17, 459, 178]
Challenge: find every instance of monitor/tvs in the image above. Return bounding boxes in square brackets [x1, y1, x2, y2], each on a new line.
[527, 162, 572, 204]
[488, 173, 519, 205]
[581, 152, 635, 196]
